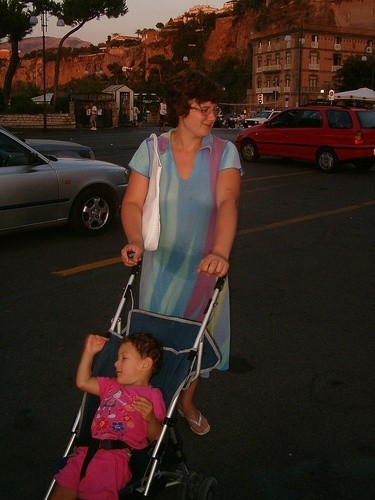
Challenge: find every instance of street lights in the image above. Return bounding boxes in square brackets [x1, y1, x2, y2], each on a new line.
[29, 6, 65, 130]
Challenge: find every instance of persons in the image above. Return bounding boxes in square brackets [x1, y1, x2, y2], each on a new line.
[158, 97, 168, 132]
[90, 103, 98, 131]
[51, 332, 166, 500]
[121, 67, 242, 435]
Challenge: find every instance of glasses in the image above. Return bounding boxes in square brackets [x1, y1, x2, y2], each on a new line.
[190, 106, 221, 116]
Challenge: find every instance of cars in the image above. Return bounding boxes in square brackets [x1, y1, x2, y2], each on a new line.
[22, 136, 95, 160]
[245, 109, 280, 129]
[235, 107, 374, 176]
[0, 128, 130, 241]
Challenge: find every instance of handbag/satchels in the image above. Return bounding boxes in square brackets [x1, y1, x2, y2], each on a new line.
[141, 132, 163, 251]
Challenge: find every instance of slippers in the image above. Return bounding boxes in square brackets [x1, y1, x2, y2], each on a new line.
[179, 408, 209, 435]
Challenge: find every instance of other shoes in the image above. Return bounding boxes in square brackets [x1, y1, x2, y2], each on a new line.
[91, 127, 94, 130]
[92, 128, 97, 130]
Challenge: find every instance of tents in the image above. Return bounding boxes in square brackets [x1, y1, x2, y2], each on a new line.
[328, 88, 375, 106]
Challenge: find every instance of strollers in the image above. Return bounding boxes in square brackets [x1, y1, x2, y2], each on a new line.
[45, 253, 226, 499]
